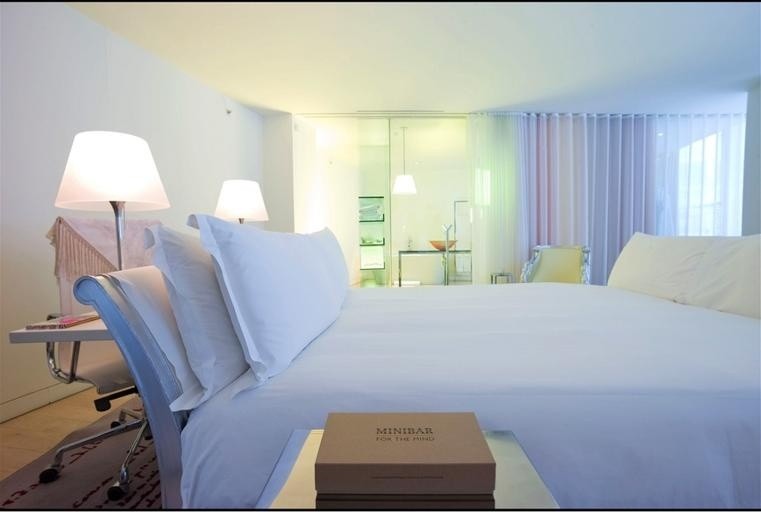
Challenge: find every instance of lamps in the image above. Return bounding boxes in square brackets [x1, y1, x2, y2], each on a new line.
[394, 127, 417, 195]
[56, 132, 171, 271]
[213, 179, 269, 224]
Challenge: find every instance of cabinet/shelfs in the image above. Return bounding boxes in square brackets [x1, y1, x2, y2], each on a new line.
[359, 195, 386, 270]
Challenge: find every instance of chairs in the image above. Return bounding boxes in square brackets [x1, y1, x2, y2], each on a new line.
[520, 245, 592, 285]
[10, 217, 153, 503]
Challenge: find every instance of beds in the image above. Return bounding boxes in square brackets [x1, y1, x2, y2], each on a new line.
[74, 267, 760, 512]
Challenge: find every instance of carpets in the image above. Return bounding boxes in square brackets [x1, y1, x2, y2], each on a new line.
[0, 396, 161, 511]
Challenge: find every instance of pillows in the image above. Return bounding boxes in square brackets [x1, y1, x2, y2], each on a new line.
[607, 232, 761, 318]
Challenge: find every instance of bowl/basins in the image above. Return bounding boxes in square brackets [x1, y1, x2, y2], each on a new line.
[430, 240, 458, 249]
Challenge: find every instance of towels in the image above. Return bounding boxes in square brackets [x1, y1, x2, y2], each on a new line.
[358, 205, 381, 221]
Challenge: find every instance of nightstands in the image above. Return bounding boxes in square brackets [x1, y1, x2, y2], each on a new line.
[254, 428, 561, 511]
